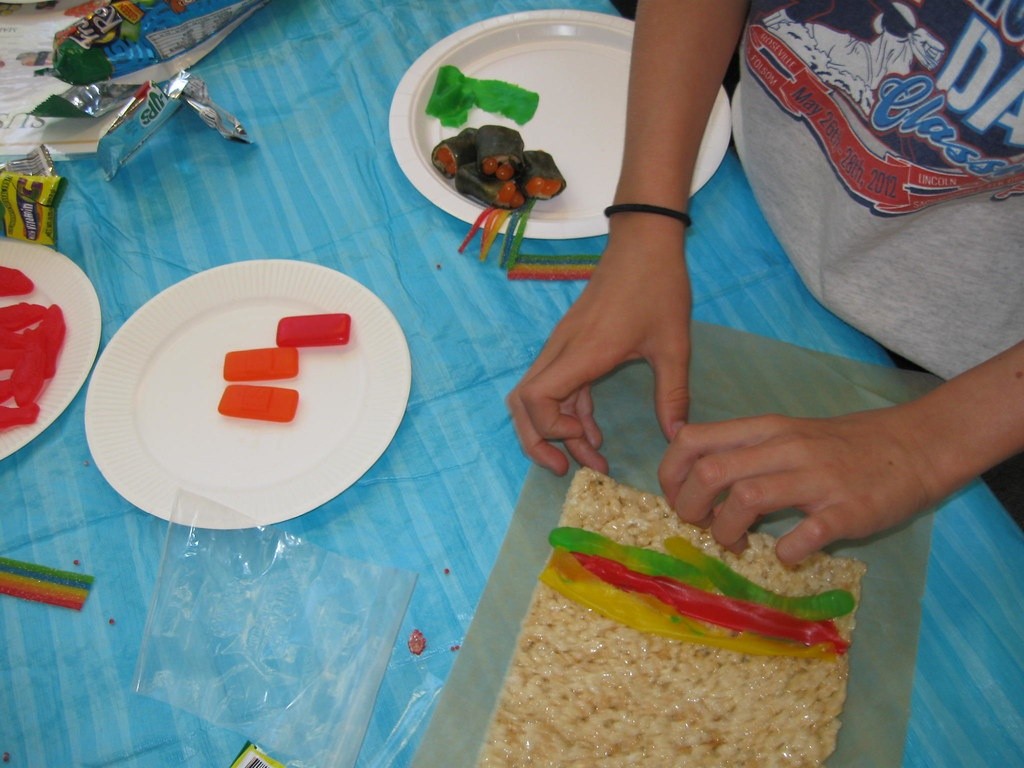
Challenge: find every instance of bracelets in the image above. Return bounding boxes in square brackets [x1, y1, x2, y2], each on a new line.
[604, 203, 692, 227]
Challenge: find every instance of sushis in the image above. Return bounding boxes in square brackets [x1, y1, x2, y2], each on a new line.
[432, 126, 567, 210]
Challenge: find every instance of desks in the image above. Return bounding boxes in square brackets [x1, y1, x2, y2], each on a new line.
[0, 1, 1024, 768]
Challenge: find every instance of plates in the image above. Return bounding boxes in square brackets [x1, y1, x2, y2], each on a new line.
[0, 236, 102, 462]
[83, 258, 412, 530]
[387, 8, 733, 241]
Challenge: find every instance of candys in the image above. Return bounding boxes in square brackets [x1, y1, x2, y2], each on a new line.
[539, 526, 855, 661]
[217, 313, 352, 423]
[0, 267, 67, 433]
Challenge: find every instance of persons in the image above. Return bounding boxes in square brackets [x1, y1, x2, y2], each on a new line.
[505, 0, 1024, 572]
